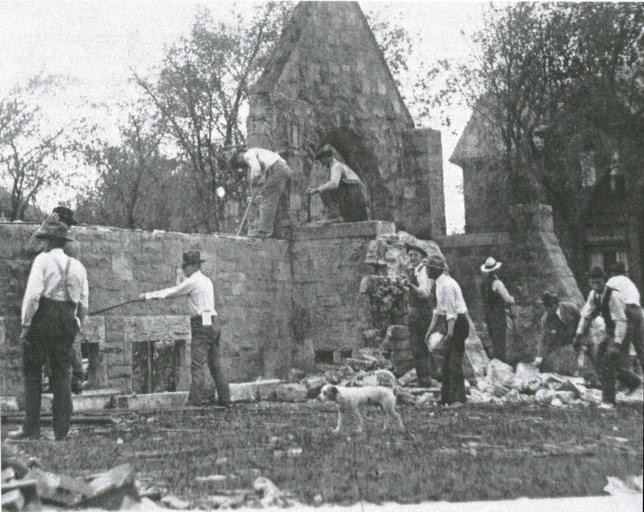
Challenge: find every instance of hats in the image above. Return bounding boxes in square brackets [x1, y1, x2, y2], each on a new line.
[35, 207, 77, 241]
[480, 257, 502, 273]
[176, 251, 207, 267]
[405, 239, 448, 270]
[538, 262, 629, 304]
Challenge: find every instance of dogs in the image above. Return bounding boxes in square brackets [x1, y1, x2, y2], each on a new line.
[315, 382, 408, 435]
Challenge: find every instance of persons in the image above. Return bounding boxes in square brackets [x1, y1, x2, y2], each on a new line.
[132, 247, 232, 407]
[4, 217, 90, 441]
[228, 147, 296, 242]
[479, 253, 515, 363]
[604, 261, 644, 376]
[530, 288, 644, 397]
[389, 239, 442, 388]
[305, 141, 370, 226]
[571, 264, 641, 409]
[419, 252, 472, 412]
[23, 205, 86, 395]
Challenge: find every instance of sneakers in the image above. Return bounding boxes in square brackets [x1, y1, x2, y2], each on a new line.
[320, 216, 344, 224]
[596, 378, 641, 408]
[249, 230, 268, 239]
[7, 428, 39, 440]
